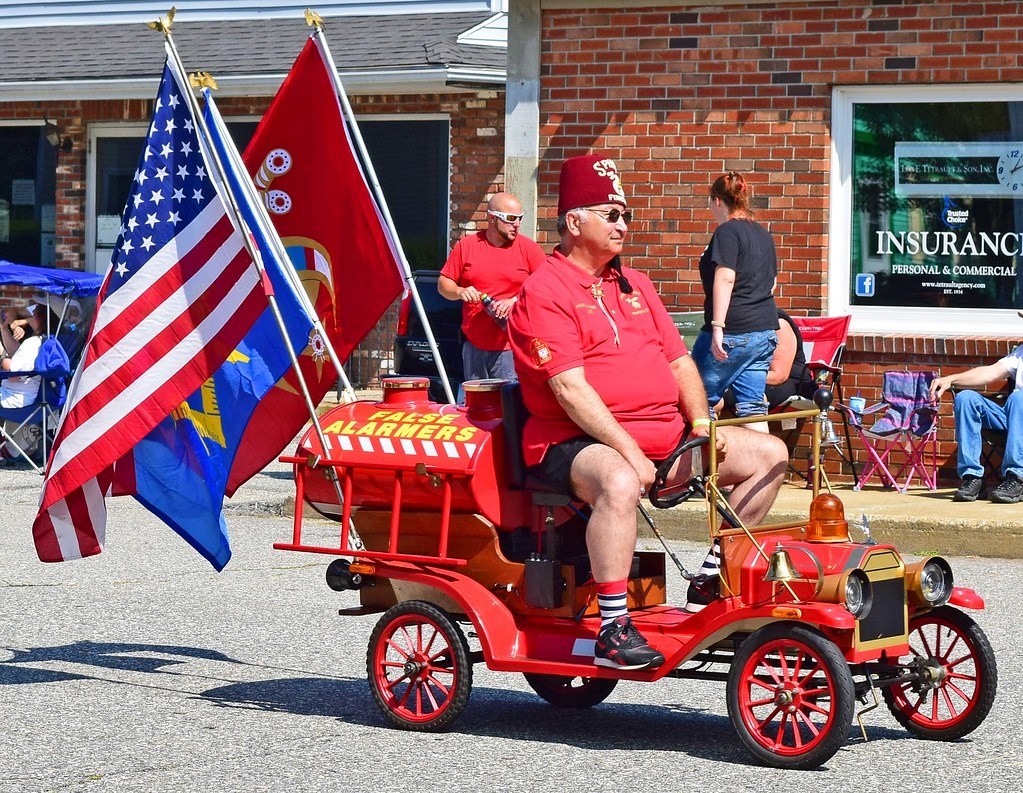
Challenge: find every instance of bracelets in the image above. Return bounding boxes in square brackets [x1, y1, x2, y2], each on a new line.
[692, 418, 712, 427]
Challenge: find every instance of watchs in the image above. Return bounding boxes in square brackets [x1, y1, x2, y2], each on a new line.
[1, 353, 12, 360]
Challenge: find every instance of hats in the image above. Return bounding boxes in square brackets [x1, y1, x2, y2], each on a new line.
[557, 154, 628, 214]
[33, 294, 70, 322]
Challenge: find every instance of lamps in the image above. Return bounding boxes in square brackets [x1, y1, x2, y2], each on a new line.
[44, 117, 73, 153]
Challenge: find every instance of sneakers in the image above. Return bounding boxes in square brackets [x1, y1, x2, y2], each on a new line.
[991, 474, 1023, 503]
[954, 477, 984, 501]
[684, 574, 720, 611]
[594, 614, 665, 670]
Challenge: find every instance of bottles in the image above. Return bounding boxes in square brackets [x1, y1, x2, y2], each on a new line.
[481, 293, 509, 330]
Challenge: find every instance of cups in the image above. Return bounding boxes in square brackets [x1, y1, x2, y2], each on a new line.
[849, 396, 866, 425]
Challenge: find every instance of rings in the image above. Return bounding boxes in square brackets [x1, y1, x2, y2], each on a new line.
[640, 487, 645, 493]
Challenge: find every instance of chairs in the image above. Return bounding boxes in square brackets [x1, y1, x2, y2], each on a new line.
[726, 312, 856, 490]
[499, 383, 587, 564]
[836, 368, 942, 495]
[943, 345, 1021, 487]
[0, 333, 84, 476]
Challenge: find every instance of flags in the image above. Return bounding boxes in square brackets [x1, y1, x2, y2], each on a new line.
[132, 88, 315, 576]
[32, 40, 277, 563]
[221, 34, 407, 499]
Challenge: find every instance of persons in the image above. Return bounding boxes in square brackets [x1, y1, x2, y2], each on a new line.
[505, 157, 789, 672]
[436, 191, 547, 381]
[713, 308, 818, 461]
[690, 169, 779, 434]
[0, 294, 69, 427]
[10, 298, 84, 341]
[929, 308, 1023, 505]
[5, 309, 37, 344]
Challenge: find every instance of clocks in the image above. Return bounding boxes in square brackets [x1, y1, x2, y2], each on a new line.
[996, 147, 1023, 191]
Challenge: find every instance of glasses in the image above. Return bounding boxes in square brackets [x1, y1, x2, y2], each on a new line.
[487, 209, 524, 223]
[579, 208, 633, 225]
